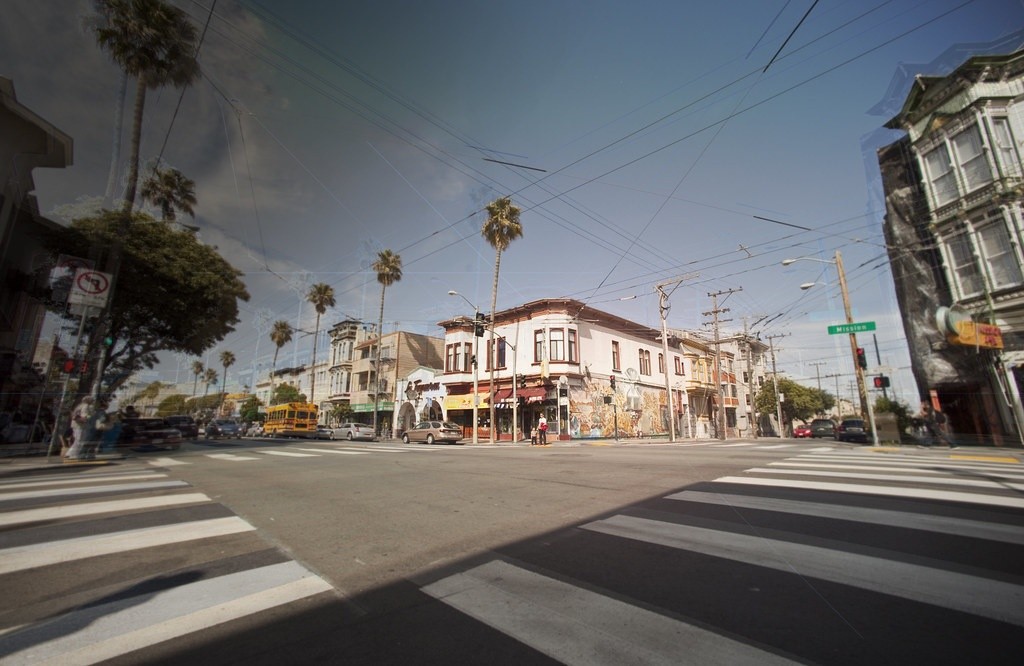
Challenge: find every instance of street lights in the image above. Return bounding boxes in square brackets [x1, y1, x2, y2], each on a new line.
[449, 291, 479, 444]
[48, 220, 200, 458]
[783, 258, 879, 445]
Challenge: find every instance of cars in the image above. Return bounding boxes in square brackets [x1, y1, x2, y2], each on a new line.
[332, 423, 376, 442]
[794, 425, 811, 438]
[317, 425, 333, 439]
[401, 420, 462, 444]
[121, 418, 181, 453]
[205, 419, 244, 440]
[166, 416, 198, 441]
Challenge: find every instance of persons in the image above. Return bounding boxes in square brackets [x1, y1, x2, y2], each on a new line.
[917, 400, 959, 450]
[531, 428, 538, 445]
[537, 413, 547, 445]
[65, 396, 140, 460]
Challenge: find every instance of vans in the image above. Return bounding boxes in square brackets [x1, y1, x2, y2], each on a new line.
[810, 418, 839, 438]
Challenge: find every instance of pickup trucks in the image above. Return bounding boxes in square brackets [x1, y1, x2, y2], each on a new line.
[838, 420, 868, 441]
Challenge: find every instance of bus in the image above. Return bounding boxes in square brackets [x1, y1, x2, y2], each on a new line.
[262, 402, 319, 440]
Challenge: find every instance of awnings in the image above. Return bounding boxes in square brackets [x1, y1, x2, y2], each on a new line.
[488, 386, 553, 403]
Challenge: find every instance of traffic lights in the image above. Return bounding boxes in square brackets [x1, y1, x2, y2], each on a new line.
[604, 397, 610, 403]
[521, 375, 527, 388]
[874, 376, 891, 388]
[474, 311, 484, 337]
[856, 347, 867, 368]
[63, 358, 75, 372]
[610, 375, 615, 388]
